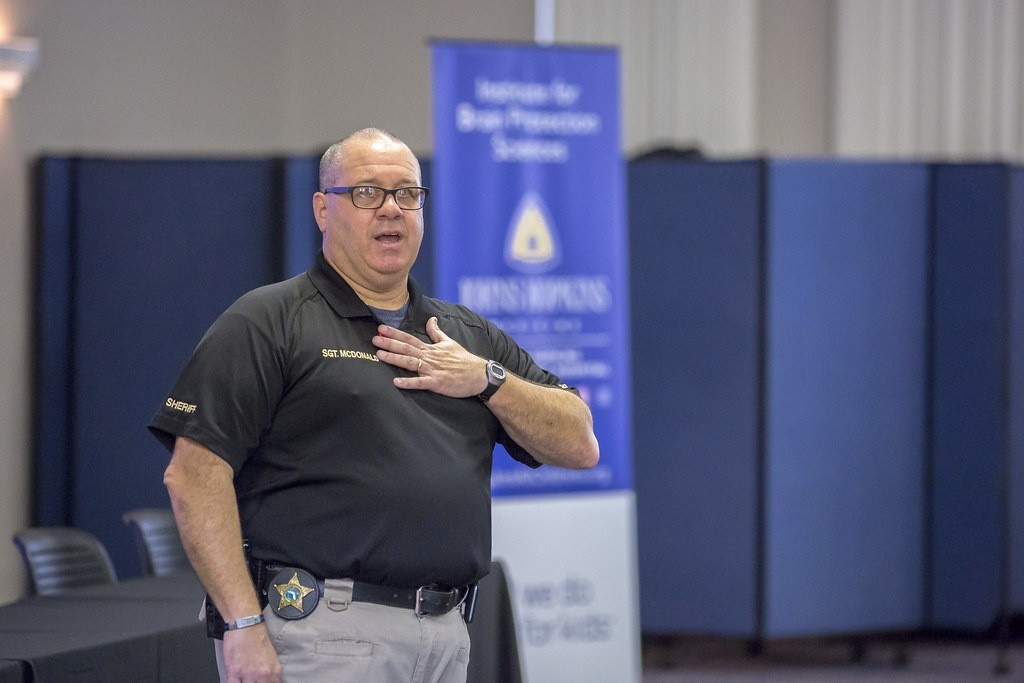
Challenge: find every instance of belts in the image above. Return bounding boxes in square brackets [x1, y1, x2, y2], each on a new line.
[262, 571, 465, 618]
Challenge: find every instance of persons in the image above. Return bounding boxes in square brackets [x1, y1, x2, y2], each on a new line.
[147, 126, 601, 683]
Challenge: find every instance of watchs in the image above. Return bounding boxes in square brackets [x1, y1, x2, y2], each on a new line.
[476, 359, 507, 403]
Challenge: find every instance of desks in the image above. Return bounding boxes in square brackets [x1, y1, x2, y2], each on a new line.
[0, 561, 523, 683]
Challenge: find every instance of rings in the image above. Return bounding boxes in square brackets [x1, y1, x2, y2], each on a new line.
[417, 358, 423, 373]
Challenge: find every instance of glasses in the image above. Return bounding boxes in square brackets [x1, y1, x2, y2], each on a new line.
[323, 186, 430, 210]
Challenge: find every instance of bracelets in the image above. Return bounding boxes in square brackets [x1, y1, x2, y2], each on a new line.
[223, 614, 265, 631]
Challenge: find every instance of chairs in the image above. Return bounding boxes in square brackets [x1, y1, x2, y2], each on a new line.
[11, 529, 117, 594]
[121, 511, 189, 576]
